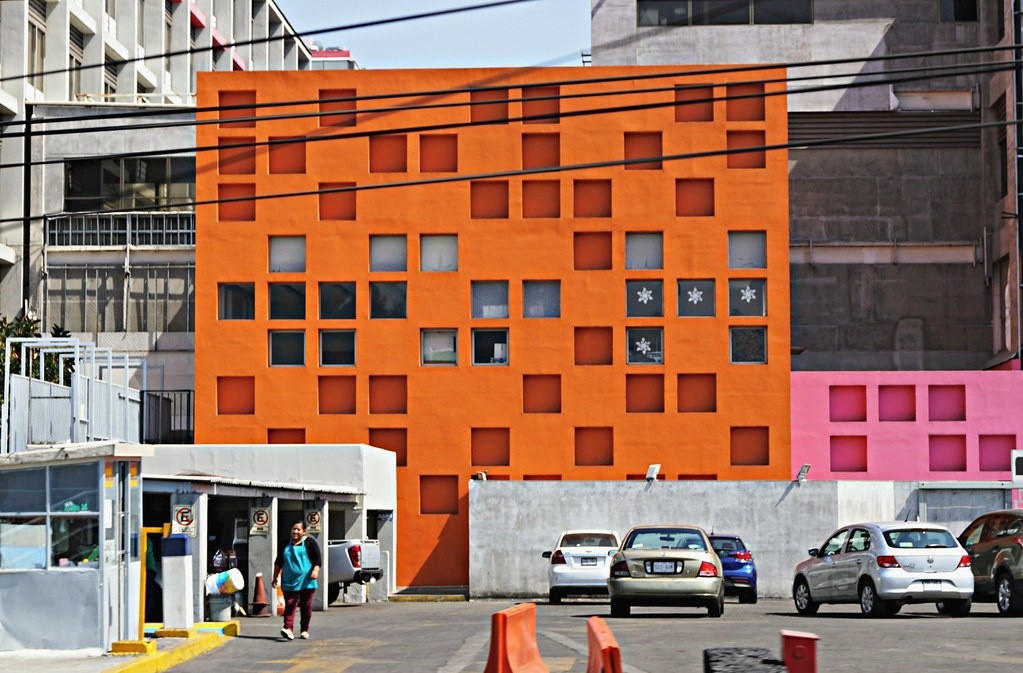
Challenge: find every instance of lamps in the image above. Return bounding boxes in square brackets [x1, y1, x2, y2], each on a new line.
[646, 462, 661, 483]
[796, 464, 811, 485]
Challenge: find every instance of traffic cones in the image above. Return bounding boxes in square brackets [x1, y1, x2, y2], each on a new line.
[247, 575, 272, 617]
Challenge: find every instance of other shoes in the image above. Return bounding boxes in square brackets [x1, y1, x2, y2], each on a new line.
[281, 628, 294, 640]
[301, 631, 309, 639]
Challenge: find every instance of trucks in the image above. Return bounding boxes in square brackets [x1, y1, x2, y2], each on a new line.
[207, 517, 383, 606]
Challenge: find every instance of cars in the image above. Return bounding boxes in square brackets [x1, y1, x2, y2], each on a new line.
[675, 533, 758, 604]
[606, 524, 727, 618]
[542, 530, 622, 603]
[936, 508, 1023, 616]
[792, 521, 974, 618]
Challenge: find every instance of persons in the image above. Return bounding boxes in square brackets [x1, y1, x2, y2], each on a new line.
[272, 520, 322, 640]
[145, 537, 157, 621]
